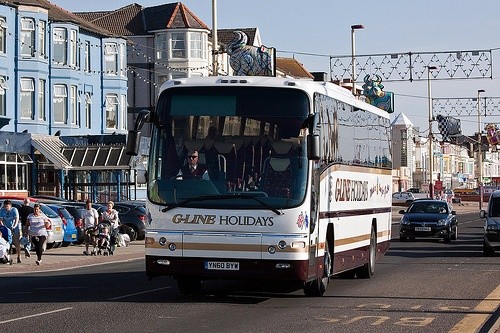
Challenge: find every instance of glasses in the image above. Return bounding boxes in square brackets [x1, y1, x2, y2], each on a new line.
[187, 156, 198, 159]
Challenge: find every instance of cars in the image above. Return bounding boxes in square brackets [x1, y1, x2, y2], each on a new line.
[0, 189, 147, 250]
[479, 188, 500, 257]
[392, 188, 454, 207]
[399, 200, 459, 243]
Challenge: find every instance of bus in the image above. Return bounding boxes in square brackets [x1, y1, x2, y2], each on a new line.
[124, 75, 393, 299]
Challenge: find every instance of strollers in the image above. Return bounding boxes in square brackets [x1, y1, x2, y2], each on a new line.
[88, 220, 116, 256]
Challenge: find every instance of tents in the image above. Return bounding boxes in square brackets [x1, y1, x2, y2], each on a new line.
[31, 137, 72, 169]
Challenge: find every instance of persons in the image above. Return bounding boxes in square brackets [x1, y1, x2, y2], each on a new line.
[441, 190, 447, 201]
[0, 200, 22, 264]
[176, 149, 210, 180]
[24, 204, 51, 266]
[98, 201, 119, 254]
[95, 227, 110, 247]
[438, 205, 447, 213]
[20, 231, 31, 258]
[80, 200, 99, 255]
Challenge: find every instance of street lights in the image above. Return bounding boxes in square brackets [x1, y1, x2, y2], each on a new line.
[351, 24, 365, 95]
[425, 66, 437, 198]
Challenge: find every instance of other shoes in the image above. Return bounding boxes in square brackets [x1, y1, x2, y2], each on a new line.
[17, 260, 21, 263]
[24, 248, 30, 257]
[38, 260, 42, 262]
[91, 251, 96, 254]
[10, 260, 13, 265]
[36, 261, 39, 265]
[83, 251, 89, 255]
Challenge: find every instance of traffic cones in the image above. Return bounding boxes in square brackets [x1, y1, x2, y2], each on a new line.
[477, 89, 485, 210]
